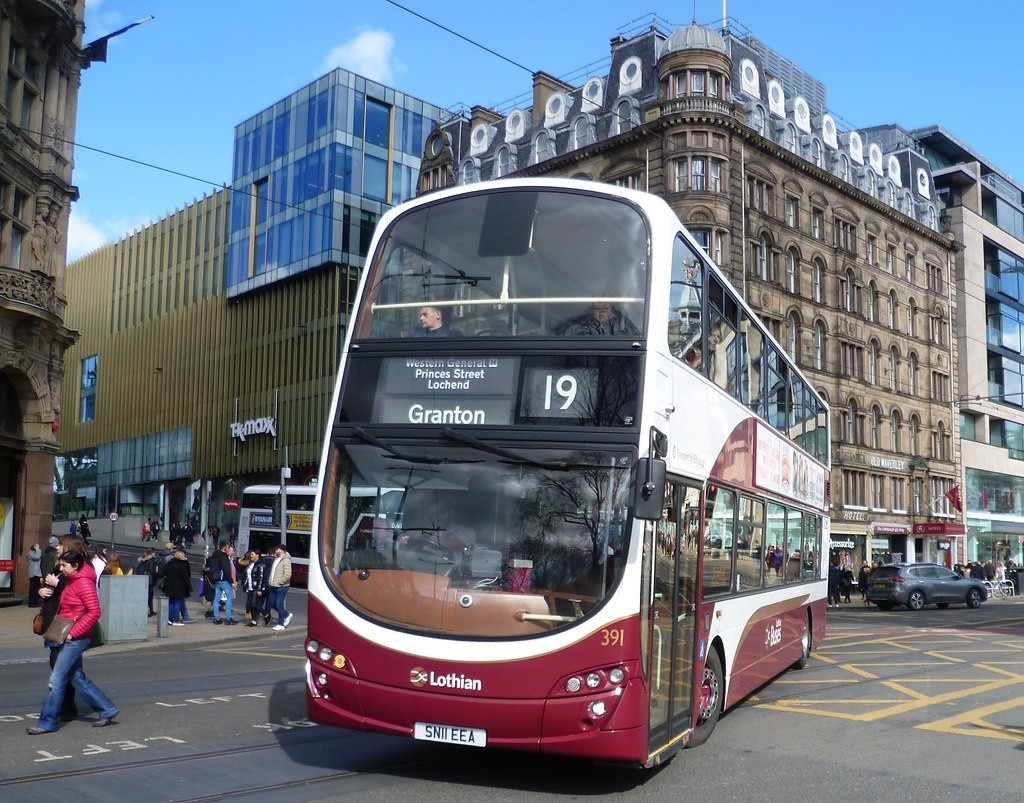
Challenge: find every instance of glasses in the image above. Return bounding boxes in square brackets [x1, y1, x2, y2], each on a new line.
[431, 509, 446, 517]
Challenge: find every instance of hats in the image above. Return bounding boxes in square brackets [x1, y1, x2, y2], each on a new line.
[49, 536, 59, 547]
[278, 544, 287, 552]
[165, 543, 177, 550]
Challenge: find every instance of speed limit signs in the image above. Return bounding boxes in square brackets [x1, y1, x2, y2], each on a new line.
[109, 512, 119, 522]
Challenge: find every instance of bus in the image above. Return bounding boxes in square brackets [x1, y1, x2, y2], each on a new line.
[235, 485, 406, 584]
[306, 177, 831, 769]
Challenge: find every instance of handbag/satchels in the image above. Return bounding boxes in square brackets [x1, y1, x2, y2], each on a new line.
[45, 615, 74, 644]
[87, 620, 105, 649]
[32, 614, 45, 635]
[197, 572, 204, 597]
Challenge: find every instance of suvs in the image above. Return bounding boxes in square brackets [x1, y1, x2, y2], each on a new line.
[866, 563, 987, 610]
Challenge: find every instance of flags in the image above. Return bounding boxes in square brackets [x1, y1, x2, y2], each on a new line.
[945, 486, 962, 512]
[80, 23, 140, 70]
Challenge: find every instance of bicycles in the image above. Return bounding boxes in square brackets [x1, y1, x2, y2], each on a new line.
[981, 574, 1011, 599]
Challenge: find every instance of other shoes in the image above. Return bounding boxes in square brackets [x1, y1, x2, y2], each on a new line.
[183, 618, 196, 623]
[172, 620, 185, 626]
[244, 613, 252, 619]
[284, 613, 293, 626]
[93, 710, 119, 727]
[829, 597, 868, 608]
[204, 610, 215, 617]
[148, 611, 157, 617]
[225, 618, 238, 625]
[26, 726, 46, 734]
[168, 620, 173, 625]
[214, 618, 223, 625]
[273, 624, 285, 630]
[264, 615, 271, 627]
[248, 620, 257, 627]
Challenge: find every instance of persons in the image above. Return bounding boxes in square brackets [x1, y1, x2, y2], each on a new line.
[828, 561, 854, 608]
[954, 559, 1022, 582]
[25, 513, 133, 734]
[786, 549, 801, 580]
[30, 207, 62, 279]
[682, 530, 691, 548]
[238, 544, 293, 631]
[942, 562, 947, 566]
[738, 535, 750, 551]
[407, 306, 463, 337]
[140, 516, 195, 549]
[980, 486, 989, 509]
[858, 560, 883, 602]
[137, 543, 196, 626]
[766, 545, 783, 571]
[657, 531, 675, 560]
[397, 498, 476, 588]
[687, 326, 721, 365]
[562, 302, 616, 337]
[200, 526, 242, 626]
[1007, 490, 1015, 511]
